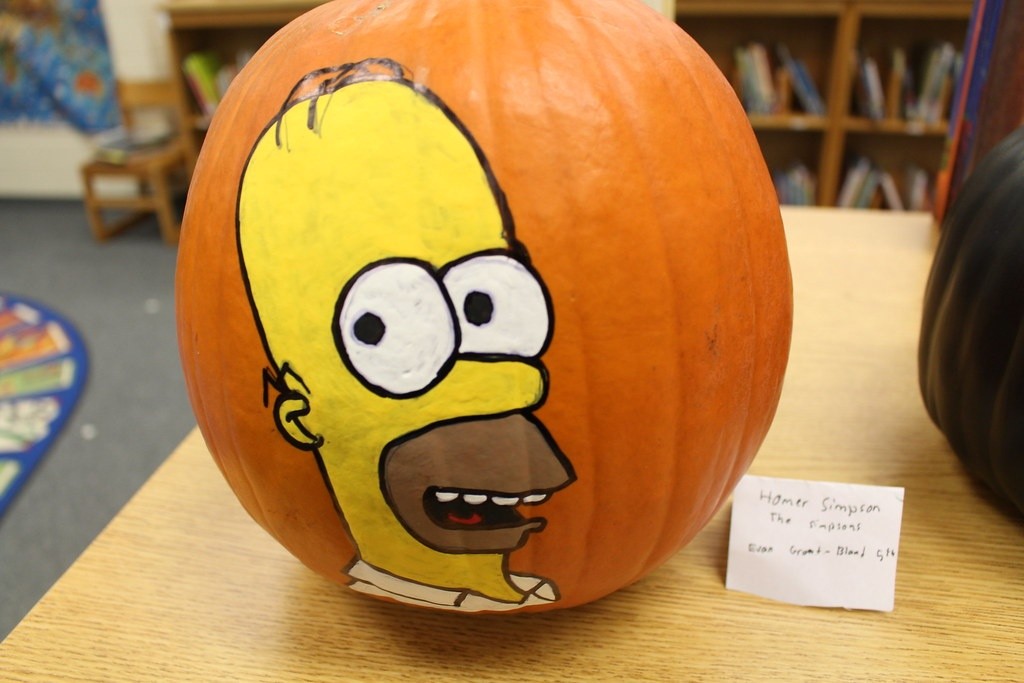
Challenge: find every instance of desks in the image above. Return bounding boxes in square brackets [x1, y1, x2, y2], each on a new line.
[2, 205, 1022, 683]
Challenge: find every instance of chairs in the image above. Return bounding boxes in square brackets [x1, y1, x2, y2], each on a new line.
[76, 74, 205, 249]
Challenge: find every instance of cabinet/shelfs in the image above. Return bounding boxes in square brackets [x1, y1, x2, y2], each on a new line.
[157, 0, 975, 215]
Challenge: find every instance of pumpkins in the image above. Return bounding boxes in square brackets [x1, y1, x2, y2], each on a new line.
[915, 127, 1023, 525]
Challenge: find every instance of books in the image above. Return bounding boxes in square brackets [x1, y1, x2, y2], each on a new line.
[854, 42, 962, 125]
[734, 42, 824, 115]
[771, 164, 817, 205]
[181, 48, 254, 118]
[838, 156, 929, 212]
